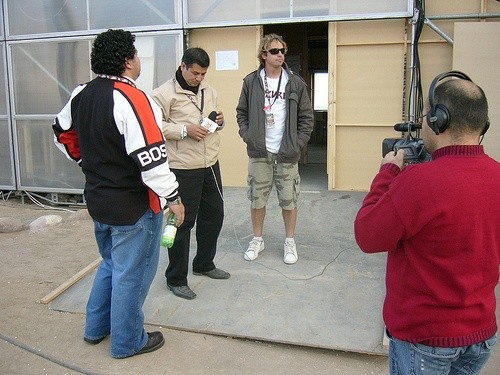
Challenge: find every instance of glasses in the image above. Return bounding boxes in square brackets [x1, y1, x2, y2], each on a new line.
[417, 114, 428, 124]
[263, 48, 285, 55]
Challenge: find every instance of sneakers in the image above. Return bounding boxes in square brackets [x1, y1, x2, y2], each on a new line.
[244, 238, 265, 261]
[283, 243, 298, 264]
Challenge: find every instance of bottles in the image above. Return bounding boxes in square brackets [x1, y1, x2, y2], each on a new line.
[159, 210, 182, 248]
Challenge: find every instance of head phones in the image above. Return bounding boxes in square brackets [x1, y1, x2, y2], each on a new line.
[426, 70, 491, 135]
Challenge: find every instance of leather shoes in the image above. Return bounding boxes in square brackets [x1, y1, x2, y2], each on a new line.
[113, 331, 165, 359]
[166, 284, 196, 300]
[194, 268, 231, 278]
[84, 337, 105, 344]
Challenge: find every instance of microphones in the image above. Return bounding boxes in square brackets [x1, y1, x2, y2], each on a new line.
[394, 122, 422, 131]
[197, 111, 218, 142]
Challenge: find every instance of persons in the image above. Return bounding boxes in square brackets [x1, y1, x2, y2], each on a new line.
[235, 33, 316, 265]
[353, 78, 500, 375]
[49, 29, 187, 361]
[149, 46, 231, 301]
